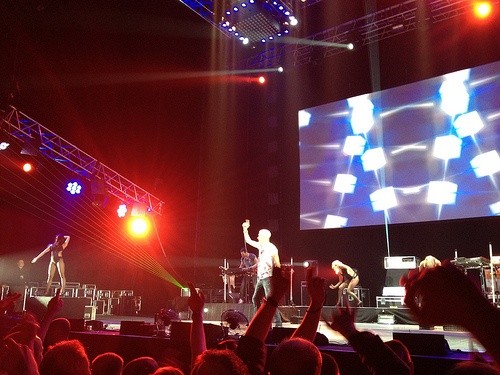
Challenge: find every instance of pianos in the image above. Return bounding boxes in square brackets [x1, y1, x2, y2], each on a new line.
[219, 266, 257, 304]
[448, 256, 490, 297]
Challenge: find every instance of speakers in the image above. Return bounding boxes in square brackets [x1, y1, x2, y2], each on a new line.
[118, 321, 166, 336]
[273, 326, 329, 347]
[301, 281, 310, 306]
[391, 333, 451, 357]
[169, 322, 228, 340]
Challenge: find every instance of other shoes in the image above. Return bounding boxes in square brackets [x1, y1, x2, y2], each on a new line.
[354, 301, 362, 306]
[238, 299, 243, 304]
[275, 322, 282, 327]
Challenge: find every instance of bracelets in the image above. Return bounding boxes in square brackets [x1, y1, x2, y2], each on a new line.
[263, 296, 278, 309]
[192, 312, 203, 318]
[306, 307, 323, 312]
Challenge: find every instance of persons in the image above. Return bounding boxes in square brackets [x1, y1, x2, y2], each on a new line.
[31, 234, 70, 297]
[13, 258, 28, 285]
[328, 260, 363, 306]
[0, 256, 500, 375]
[238, 248, 259, 304]
[242, 220, 282, 327]
